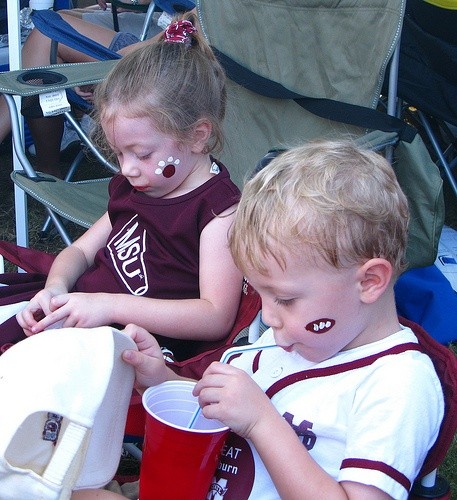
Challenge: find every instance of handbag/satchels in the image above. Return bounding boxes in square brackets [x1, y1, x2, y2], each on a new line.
[392, 131, 445, 272]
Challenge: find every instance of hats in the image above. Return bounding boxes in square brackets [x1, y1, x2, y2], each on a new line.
[0, 326, 140, 500]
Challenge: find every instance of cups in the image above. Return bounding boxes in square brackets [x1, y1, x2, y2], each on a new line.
[138, 380, 231, 499]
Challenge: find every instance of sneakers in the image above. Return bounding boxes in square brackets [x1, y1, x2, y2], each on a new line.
[29, 114, 101, 157]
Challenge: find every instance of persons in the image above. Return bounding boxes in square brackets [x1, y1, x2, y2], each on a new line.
[0, 0, 200, 143]
[0, 12, 243, 357]
[69, 137, 444, 500]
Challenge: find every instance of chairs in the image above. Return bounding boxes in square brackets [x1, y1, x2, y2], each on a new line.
[0, 0, 457, 500]
